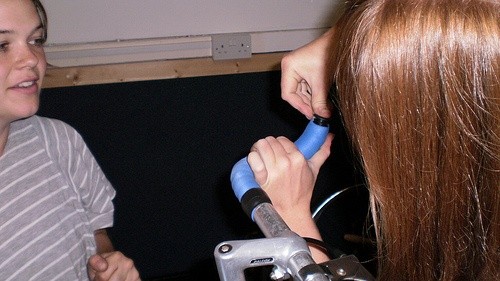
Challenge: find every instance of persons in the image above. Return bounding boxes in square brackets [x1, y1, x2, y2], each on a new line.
[248, 0, 500, 281]
[0, 0, 142, 281]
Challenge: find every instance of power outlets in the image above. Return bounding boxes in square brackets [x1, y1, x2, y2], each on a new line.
[212, 32, 253, 61]
